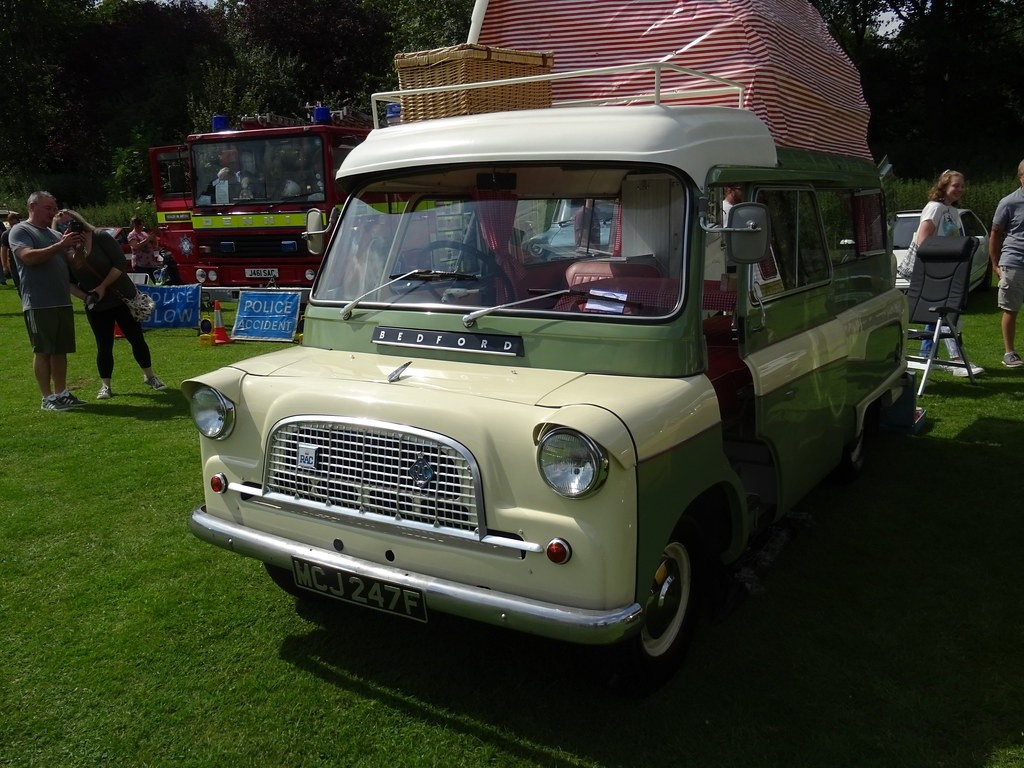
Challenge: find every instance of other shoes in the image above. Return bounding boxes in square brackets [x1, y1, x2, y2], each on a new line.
[1001, 352, 1024, 368]
[953, 360, 984, 377]
[144, 374, 166, 391]
[41, 392, 87, 412]
[97, 385, 113, 399]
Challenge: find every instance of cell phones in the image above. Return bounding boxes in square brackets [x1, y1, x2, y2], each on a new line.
[70, 222, 83, 236]
[150, 228, 155, 235]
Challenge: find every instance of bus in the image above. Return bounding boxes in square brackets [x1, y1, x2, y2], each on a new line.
[147, 126, 443, 311]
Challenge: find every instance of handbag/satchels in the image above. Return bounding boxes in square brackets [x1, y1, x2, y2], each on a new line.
[897, 210, 942, 282]
[121, 289, 155, 322]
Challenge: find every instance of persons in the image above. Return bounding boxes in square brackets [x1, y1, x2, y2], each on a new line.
[8, 191, 88, 412]
[127, 216, 159, 285]
[51, 209, 168, 399]
[989, 159, 1024, 368]
[0, 221, 8, 286]
[916, 170, 985, 377]
[573, 199, 601, 257]
[722, 186, 744, 228]
[1, 213, 22, 301]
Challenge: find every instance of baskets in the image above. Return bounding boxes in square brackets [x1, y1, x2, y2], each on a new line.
[394, 43, 554, 124]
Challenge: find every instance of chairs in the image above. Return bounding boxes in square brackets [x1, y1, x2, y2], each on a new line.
[903, 234, 979, 397]
[551, 260, 667, 316]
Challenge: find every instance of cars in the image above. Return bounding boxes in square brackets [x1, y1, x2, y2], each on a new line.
[893, 206, 993, 306]
[180, 58, 916, 668]
